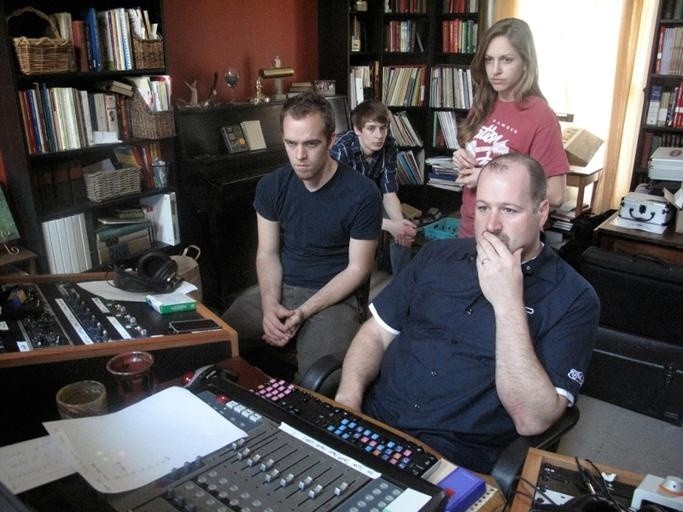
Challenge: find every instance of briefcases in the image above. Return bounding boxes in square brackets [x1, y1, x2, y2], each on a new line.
[618, 191, 673, 225]
[648, 147, 683, 182]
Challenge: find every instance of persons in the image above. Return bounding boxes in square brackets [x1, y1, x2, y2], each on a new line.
[218, 92, 385, 398]
[332, 151, 602, 478]
[325, 100, 417, 283]
[450, 17, 572, 239]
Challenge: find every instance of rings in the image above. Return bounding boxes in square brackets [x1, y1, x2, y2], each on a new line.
[481, 258, 490, 264]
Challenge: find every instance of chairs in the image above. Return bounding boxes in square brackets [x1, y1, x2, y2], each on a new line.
[299, 348, 581, 493]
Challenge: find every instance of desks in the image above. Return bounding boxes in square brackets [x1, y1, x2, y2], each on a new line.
[569, 162, 602, 226]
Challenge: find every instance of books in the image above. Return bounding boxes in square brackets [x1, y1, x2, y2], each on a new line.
[219, 124, 247, 154]
[549, 201, 592, 233]
[239, 119, 267, 151]
[14, 8, 181, 278]
[348, 1, 487, 192]
[631, 1, 682, 181]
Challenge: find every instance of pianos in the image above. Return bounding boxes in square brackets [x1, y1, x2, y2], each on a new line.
[172, 98, 353, 281]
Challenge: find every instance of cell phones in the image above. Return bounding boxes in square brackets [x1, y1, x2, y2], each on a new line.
[169, 318, 222, 334]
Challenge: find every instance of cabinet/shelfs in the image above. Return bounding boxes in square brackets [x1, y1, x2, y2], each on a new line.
[580, 0, 683, 428]
[1, 1, 181, 274]
[346, 0, 523, 267]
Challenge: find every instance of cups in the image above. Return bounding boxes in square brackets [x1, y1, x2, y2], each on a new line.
[106, 350, 156, 404]
[56, 379, 107, 421]
[151, 159, 167, 189]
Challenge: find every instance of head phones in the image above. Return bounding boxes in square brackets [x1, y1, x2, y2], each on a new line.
[553, 493, 668, 512]
[111, 249, 185, 294]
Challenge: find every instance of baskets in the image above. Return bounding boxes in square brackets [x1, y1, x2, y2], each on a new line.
[6, 6, 79, 77]
[82, 161, 143, 203]
[127, 80, 177, 140]
[131, 30, 166, 70]
[423, 217, 461, 241]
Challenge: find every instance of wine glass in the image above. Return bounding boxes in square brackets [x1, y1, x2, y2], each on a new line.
[222, 67, 241, 103]
